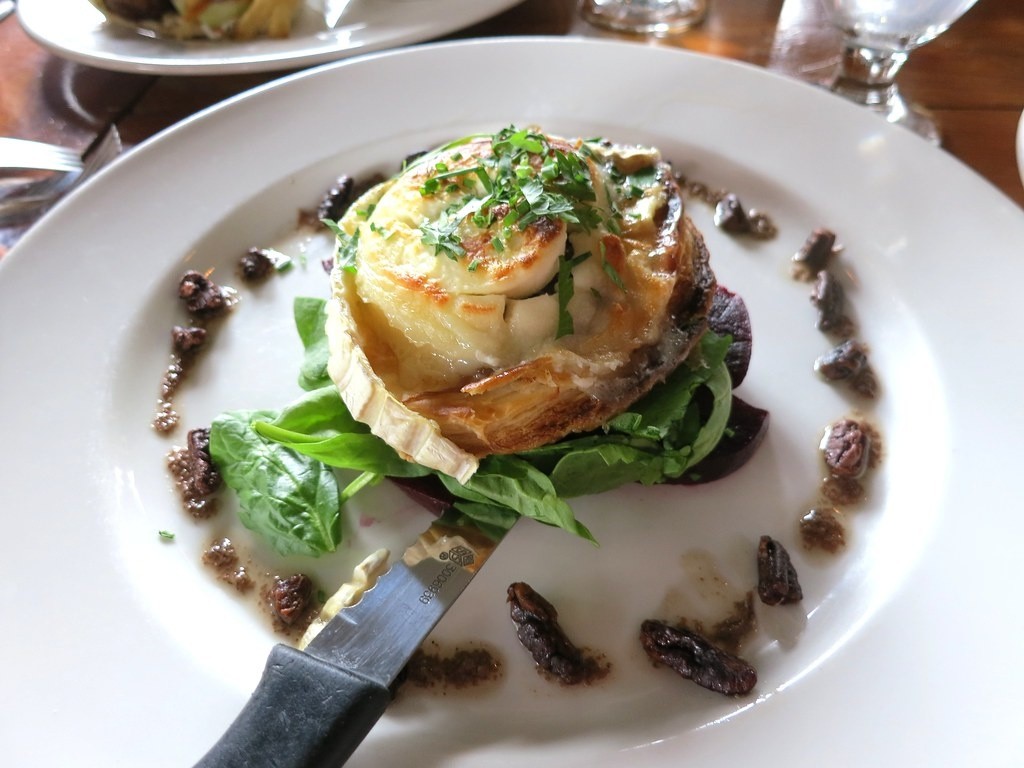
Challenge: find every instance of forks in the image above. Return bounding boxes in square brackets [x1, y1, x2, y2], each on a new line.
[0, 134, 80, 175]
[1, 124, 124, 227]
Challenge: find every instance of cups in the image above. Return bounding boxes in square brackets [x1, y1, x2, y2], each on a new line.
[580, 0, 710, 36]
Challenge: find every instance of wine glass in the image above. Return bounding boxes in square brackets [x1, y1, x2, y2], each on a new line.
[805, 1, 985, 144]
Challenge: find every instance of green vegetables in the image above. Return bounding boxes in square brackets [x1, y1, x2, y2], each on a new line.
[210, 292, 734, 562]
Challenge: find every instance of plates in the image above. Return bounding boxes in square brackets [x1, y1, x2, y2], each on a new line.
[0, 35, 1024, 768]
[15, 0, 525, 81]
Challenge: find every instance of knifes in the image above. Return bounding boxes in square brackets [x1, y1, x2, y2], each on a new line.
[194, 504, 521, 768]
